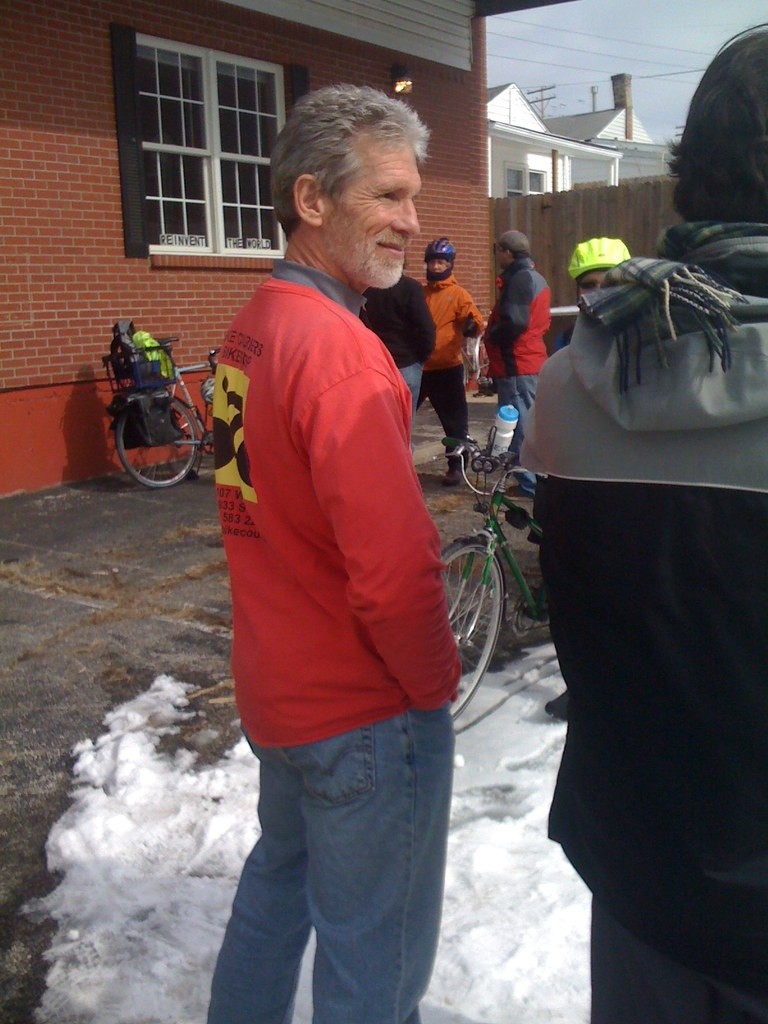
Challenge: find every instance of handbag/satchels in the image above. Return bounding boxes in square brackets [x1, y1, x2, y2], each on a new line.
[107, 385, 186, 448]
[107, 320, 175, 386]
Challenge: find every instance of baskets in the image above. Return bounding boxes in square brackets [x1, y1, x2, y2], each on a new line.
[102, 344, 177, 393]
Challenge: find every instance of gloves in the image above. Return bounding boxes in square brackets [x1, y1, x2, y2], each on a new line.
[463, 312, 479, 338]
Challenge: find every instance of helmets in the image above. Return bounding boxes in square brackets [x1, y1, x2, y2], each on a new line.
[423, 238, 456, 283]
[200, 378, 215, 403]
[568, 237, 631, 279]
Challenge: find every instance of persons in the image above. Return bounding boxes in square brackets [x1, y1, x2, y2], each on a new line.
[547, 237, 632, 357]
[416, 237, 470, 488]
[360, 248, 436, 466]
[533, 23, 768, 1024]
[481, 232, 552, 502]
[204, 84, 461, 1017]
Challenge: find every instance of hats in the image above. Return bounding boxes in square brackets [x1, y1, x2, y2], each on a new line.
[498, 230, 529, 252]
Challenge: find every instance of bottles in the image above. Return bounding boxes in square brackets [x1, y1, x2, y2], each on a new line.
[490, 404, 519, 457]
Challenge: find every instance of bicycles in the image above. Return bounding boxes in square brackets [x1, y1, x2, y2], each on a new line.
[437, 425, 551, 723]
[460, 319, 490, 387]
[100, 336, 222, 488]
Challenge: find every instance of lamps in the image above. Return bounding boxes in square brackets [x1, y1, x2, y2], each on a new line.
[390, 63, 412, 94]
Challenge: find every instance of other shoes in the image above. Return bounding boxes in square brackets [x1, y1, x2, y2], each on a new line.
[443, 467, 462, 486]
[503, 484, 535, 501]
[545, 687, 571, 722]
